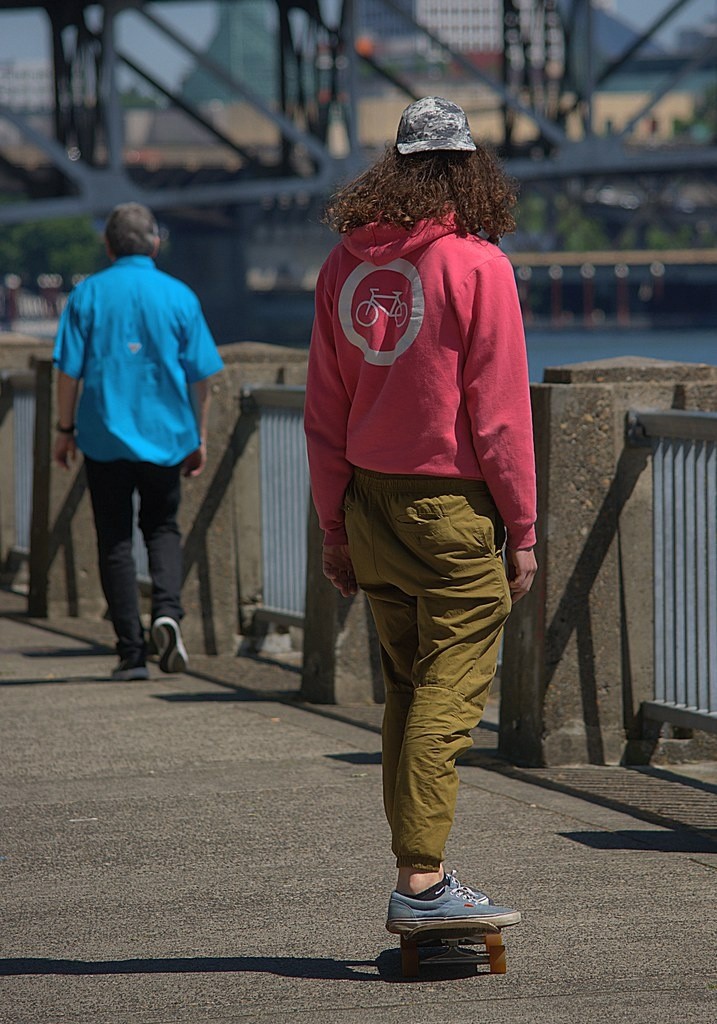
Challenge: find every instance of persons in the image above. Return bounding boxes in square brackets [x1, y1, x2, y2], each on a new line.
[52, 201, 225, 679]
[303, 97, 536, 934]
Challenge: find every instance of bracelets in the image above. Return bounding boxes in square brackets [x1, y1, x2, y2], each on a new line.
[55, 421, 76, 434]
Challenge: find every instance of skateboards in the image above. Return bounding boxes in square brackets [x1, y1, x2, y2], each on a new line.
[393, 887, 508, 975]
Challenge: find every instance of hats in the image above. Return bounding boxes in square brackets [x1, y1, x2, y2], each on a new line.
[396, 97, 477, 155]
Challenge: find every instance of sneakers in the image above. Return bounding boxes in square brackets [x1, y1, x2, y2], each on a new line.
[111, 666, 147, 680]
[150, 616, 190, 674]
[386, 870, 521, 934]
